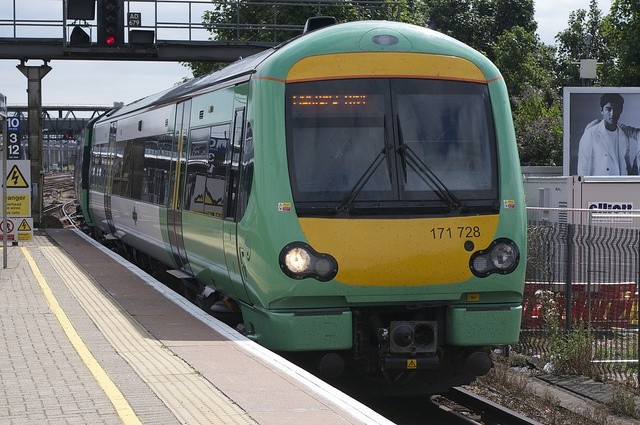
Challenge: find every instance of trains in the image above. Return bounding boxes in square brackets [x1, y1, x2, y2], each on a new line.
[76, 19, 527, 387]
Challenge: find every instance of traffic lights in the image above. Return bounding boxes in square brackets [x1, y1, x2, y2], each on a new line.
[97, 0, 123, 46]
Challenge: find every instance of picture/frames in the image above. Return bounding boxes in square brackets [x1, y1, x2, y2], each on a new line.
[563, 87, 640, 176]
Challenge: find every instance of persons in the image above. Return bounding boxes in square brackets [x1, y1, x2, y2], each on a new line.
[584, 119, 640, 175]
[577, 93, 630, 176]
